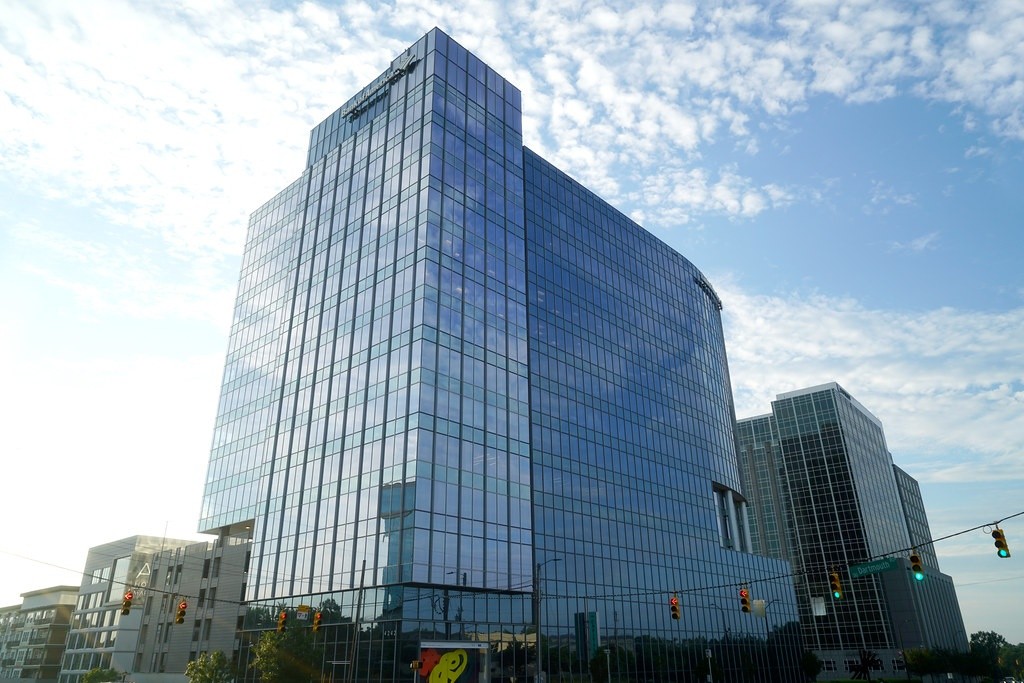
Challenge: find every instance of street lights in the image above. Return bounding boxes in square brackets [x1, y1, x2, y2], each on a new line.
[536, 558, 564, 683]
[746, 598, 784, 674]
[613, 609, 621, 683]
[707, 600, 741, 683]
[445, 571, 469, 588]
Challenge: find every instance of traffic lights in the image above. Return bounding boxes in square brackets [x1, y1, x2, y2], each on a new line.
[991, 528, 1010, 558]
[909, 553, 924, 581]
[175, 599, 187, 625]
[670, 597, 679, 620]
[739, 587, 751, 613]
[313, 611, 324, 633]
[828, 570, 843, 601]
[121, 589, 134, 616]
[277, 610, 289, 634]
